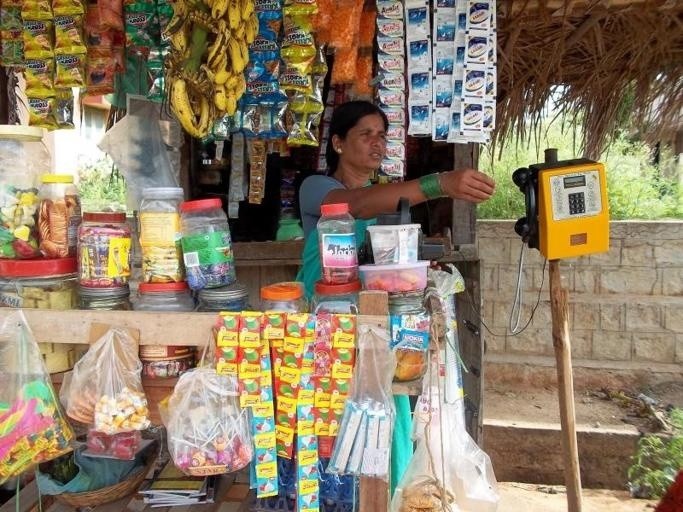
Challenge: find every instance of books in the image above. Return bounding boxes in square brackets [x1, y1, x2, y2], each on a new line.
[136, 459, 216, 508]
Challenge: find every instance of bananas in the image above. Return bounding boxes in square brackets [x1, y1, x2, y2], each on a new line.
[162, 0, 259, 138]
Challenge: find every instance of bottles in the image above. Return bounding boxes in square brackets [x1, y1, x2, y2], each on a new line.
[78, 211, 135, 289]
[77, 286, 132, 365]
[136, 186, 186, 284]
[132, 282, 199, 378]
[0, 123, 52, 259]
[191, 282, 258, 366]
[0, 261, 89, 378]
[180, 199, 237, 291]
[316, 203, 361, 284]
[276, 219, 304, 241]
[38, 175, 85, 258]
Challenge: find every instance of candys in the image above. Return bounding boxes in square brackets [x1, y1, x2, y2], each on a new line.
[0, 184, 69, 257]
[94, 388, 151, 434]
[170, 408, 253, 478]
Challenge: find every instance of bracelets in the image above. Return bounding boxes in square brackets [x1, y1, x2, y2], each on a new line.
[419, 173, 448, 200]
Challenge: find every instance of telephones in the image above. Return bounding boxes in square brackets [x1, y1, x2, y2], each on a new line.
[511, 157, 611, 261]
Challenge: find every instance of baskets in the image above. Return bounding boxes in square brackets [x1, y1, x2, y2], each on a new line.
[54, 467, 148, 507]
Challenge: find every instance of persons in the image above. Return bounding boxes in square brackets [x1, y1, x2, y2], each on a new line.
[296, 101, 496, 500]
